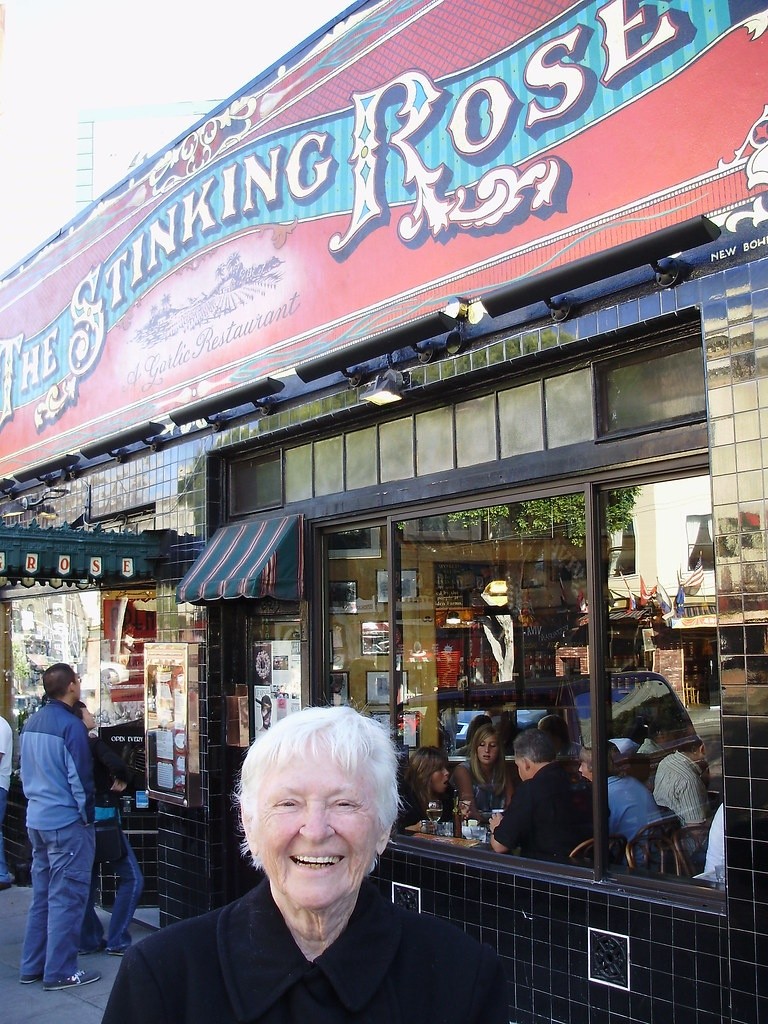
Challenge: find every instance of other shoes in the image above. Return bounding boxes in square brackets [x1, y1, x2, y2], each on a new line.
[107, 946, 131, 955]
[0, 882, 11, 890]
[78, 939, 107, 955]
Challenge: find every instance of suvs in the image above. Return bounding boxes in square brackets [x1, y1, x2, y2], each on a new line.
[381, 671, 710, 802]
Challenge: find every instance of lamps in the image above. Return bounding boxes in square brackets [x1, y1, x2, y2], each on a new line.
[0, 497, 33, 517]
[294, 311, 463, 389]
[35, 505, 59, 520]
[481, 562, 508, 607]
[169, 378, 286, 432]
[80, 422, 166, 463]
[480, 215, 723, 322]
[360, 370, 404, 407]
[13, 453, 83, 488]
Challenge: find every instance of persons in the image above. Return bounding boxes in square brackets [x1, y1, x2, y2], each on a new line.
[0, 717, 13, 892]
[20, 663, 148, 990]
[102, 708, 511, 1024]
[400, 712, 727, 888]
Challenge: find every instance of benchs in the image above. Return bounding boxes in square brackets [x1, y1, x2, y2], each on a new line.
[625, 800, 719, 879]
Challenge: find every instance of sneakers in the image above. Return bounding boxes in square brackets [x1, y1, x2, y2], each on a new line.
[20, 974, 43, 984]
[43, 969, 102, 990]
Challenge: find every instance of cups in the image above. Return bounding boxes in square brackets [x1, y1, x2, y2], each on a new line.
[462, 814, 487, 840]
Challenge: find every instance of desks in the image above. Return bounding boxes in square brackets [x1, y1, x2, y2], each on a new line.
[405, 812, 492, 851]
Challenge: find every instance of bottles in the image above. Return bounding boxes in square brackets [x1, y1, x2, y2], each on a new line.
[452, 790, 462, 838]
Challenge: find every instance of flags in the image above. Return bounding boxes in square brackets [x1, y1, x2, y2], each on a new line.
[626, 575, 685, 618]
[683, 555, 704, 587]
[577, 589, 588, 612]
[560, 579, 568, 607]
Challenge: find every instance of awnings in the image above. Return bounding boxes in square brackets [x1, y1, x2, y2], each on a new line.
[175, 513, 307, 604]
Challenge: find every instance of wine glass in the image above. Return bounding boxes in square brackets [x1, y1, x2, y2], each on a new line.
[426, 799, 443, 834]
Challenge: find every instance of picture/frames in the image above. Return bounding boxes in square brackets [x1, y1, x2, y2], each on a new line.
[370, 710, 421, 751]
[400, 514, 484, 544]
[375, 568, 419, 605]
[328, 527, 382, 560]
[360, 620, 389, 657]
[366, 670, 409, 706]
[329, 671, 350, 707]
[330, 580, 359, 615]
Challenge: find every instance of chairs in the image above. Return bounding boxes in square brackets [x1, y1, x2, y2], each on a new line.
[566, 832, 623, 869]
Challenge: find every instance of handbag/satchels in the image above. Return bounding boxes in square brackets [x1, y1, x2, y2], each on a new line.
[94, 816, 129, 863]
